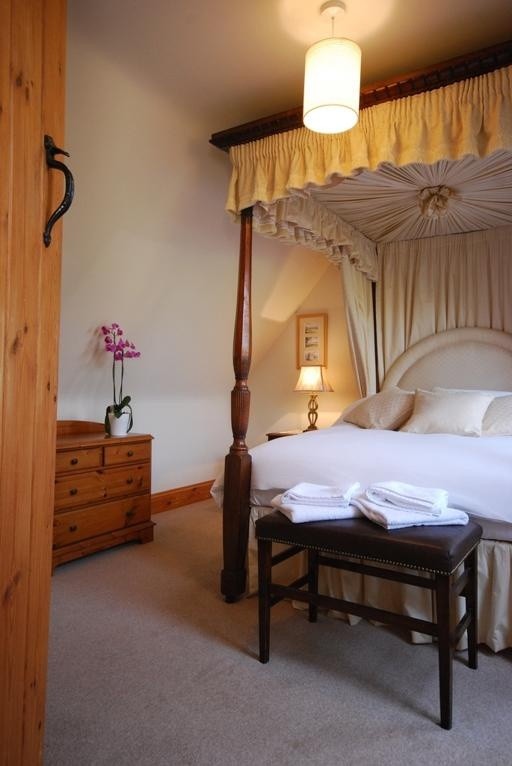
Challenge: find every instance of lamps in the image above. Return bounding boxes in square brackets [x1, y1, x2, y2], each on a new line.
[303, 0, 361, 136]
[294, 365, 334, 433]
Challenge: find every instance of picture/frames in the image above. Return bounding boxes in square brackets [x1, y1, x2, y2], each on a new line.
[296, 313, 328, 369]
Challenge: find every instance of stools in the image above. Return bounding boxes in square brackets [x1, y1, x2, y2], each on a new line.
[254, 510, 483, 730]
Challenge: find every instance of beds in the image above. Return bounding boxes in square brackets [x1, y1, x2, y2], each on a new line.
[221, 327, 512, 656]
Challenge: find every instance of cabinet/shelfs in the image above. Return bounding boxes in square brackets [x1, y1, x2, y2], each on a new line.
[53, 420, 154, 573]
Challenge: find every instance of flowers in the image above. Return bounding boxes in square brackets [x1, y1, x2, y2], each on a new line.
[101, 321, 140, 434]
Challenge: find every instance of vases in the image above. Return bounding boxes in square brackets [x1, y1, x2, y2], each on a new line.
[108, 412, 129, 436]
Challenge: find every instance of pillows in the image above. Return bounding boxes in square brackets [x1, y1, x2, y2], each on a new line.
[341, 386, 512, 437]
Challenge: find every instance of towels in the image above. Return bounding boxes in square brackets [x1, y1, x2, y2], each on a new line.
[268, 481, 469, 531]
